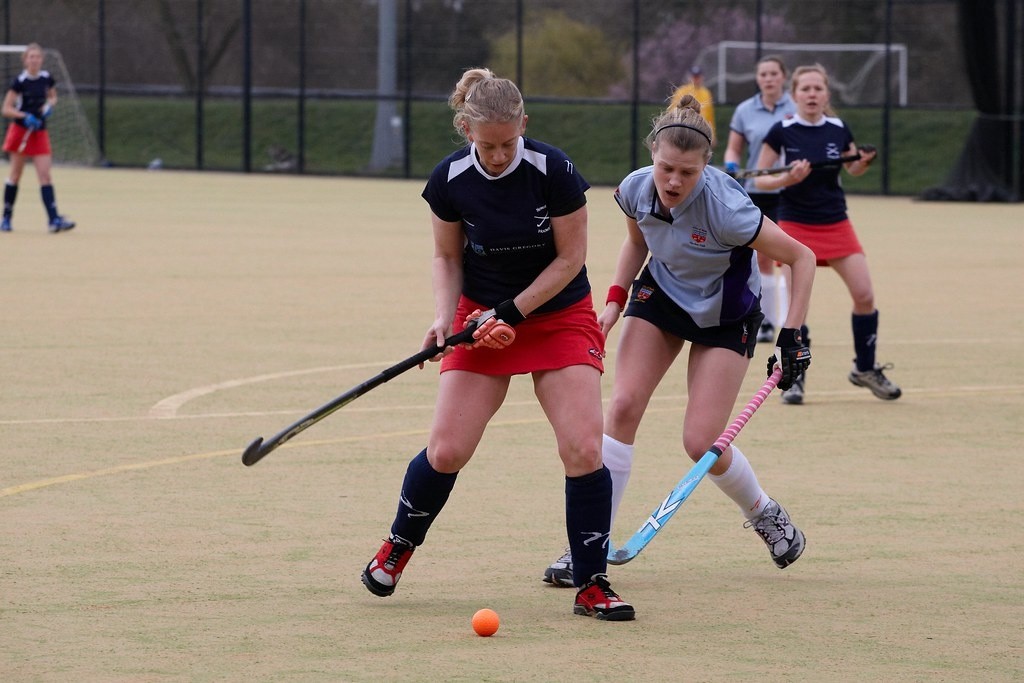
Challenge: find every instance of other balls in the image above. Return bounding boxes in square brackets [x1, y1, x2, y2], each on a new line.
[472, 609, 500, 636]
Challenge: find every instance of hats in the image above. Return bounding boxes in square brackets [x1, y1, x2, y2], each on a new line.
[690, 66, 701, 74]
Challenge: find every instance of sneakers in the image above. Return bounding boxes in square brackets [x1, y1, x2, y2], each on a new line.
[48, 216, 74, 232]
[781, 374, 805, 402]
[1, 217, 11, 231]
[361, 534, 416, 597]
[758, 321, 773, 342]
[850, 362, 902, 400]
[742, 496, 805, 568]
[541, 549, 576, 587]
[574, 573, 635, 620]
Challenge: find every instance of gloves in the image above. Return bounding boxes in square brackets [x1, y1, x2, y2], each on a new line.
[459, 300, 527, 351]
[24, 112, 42, 131]
[39, 102, 52, 119]
[766, 327, 812, 391]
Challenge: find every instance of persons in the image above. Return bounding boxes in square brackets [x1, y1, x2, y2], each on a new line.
[753, 64, 901, 404]
[540, 94, 816, 588]
[670, 67, 718, 150]
[0, 42, 76, 235]
[722, 58, 800, 343]
[360, 69, 636, 620]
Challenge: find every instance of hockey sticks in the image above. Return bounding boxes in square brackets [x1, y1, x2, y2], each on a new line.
[241, 323, 478, 467]
[606, 368, 783, 566]
[16, 112, 44, 154]
[721, 150, 869, 180]
